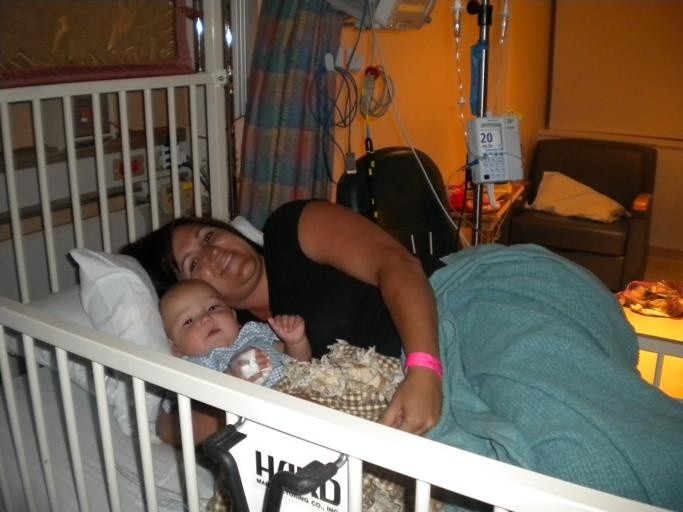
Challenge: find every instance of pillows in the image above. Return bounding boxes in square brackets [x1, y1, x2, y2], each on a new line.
[66, 216, 264, 431]
[5, 285, 116, 431]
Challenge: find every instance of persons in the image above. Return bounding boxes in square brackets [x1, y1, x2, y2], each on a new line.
[118, 197, 444, 435]
[152, 279, 312, 449]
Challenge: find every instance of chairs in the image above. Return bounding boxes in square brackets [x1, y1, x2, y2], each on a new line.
[338, 145, 462, 260]
[502, 136, 656, 293]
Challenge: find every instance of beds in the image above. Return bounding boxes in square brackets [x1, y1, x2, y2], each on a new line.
[0, 0, 682, 512]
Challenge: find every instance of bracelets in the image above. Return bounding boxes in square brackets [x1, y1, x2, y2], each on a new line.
[403, 351, 442, 381]
